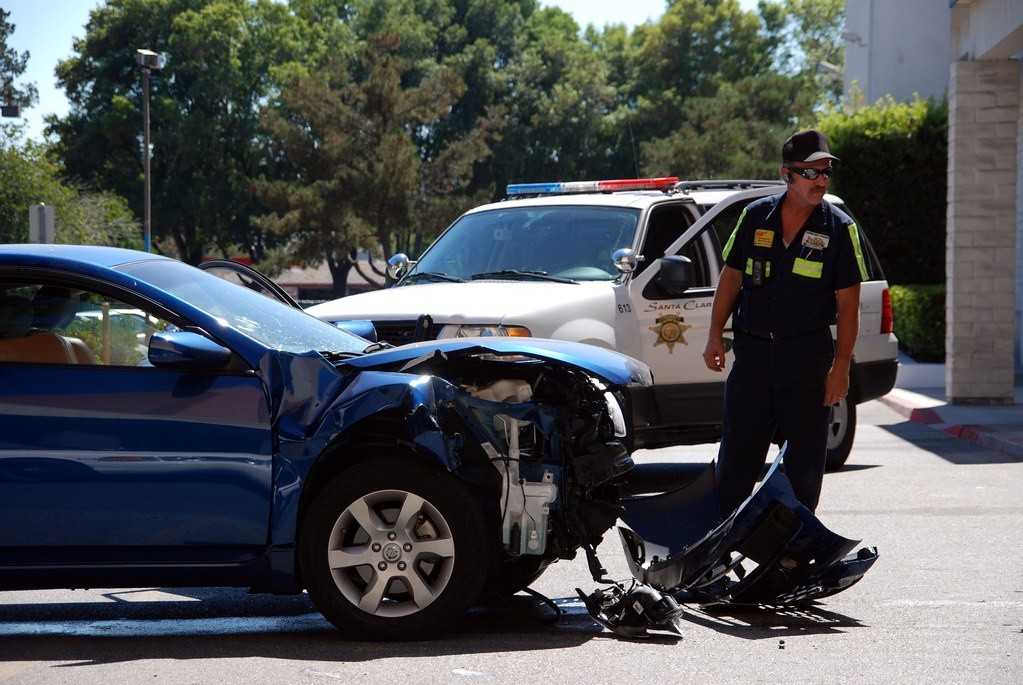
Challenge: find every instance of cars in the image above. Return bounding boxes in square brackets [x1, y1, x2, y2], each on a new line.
[0, 243, 655, 645]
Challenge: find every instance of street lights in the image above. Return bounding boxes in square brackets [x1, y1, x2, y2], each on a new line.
[135, 46, 159, 260]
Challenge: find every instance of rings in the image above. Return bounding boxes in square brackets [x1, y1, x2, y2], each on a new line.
[838, 396, 843, 400]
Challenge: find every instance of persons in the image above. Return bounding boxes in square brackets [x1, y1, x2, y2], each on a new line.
[703, 130, 875, 570]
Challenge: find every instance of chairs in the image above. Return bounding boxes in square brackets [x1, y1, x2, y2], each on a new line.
[27, 285, 96, 364]
[0, 295, 79, 363]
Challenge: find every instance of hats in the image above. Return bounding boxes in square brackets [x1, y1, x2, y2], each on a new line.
[782, 128, 840, 163]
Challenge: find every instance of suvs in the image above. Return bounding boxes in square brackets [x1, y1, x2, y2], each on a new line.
[304, 173, 901, 472]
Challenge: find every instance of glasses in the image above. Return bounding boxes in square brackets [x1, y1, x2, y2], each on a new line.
[781, 165, 832, 180]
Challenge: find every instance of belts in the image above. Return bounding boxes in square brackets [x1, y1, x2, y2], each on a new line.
[762, 332, 782, 341]
